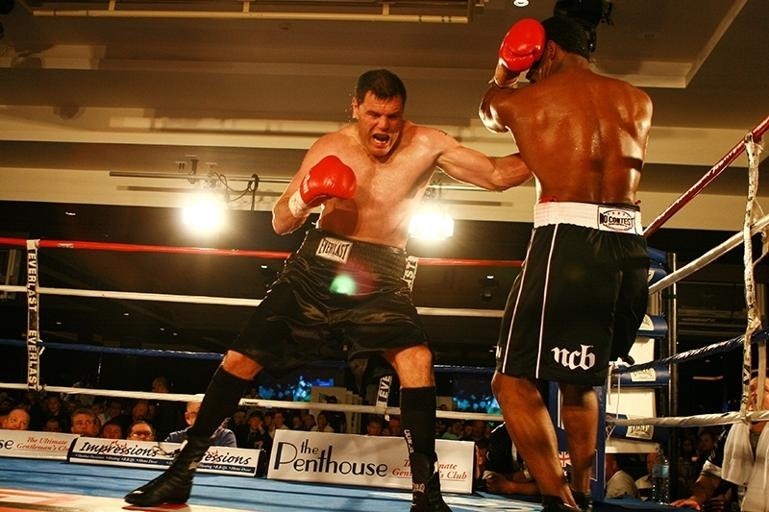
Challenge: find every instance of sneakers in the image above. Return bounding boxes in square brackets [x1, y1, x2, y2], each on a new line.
[540, 489, 593, 512]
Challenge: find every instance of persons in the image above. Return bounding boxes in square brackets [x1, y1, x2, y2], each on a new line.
[366, 418, 402, 436]
[164, 393, 344, 450]
[436, 404, 659, 499]
[2, 376, 173, 442]
[669, 368, 769, 510]
[478, 13, 654, 511]
[124, 67, 534, 512]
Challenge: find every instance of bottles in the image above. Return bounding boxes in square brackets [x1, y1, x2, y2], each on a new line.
[652, 449, 670, 503]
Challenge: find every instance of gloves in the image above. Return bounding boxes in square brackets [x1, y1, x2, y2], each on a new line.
[494, 18, 546, 87]
[289, 154, 357, 218]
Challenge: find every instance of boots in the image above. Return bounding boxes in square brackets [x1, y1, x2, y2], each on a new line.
[409, 452, 454, 512]
[124, 437, 212, 507]
